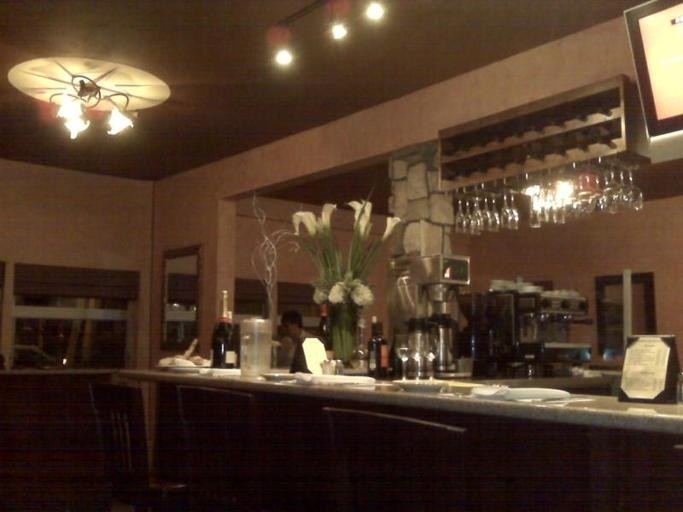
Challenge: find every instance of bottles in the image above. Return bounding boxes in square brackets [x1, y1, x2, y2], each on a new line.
[351, 313, 390, 378]
[406, 316, 439, 379]
[432, 314, 461, 373]
[209, 290, 238, 369]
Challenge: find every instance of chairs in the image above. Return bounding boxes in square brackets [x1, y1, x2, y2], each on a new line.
[88, 379, 467, 512]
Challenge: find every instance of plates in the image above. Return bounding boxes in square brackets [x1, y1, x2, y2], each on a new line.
[394, 380, 448, 394]
[261, 372, 298, 382]
[155, 364, 213, 374]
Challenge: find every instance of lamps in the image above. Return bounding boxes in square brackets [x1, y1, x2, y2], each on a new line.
[7, 54, 171, 140]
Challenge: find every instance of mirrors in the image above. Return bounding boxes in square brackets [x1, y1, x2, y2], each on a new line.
[594, 271, 657, 356]
[160, 243, 203, 353]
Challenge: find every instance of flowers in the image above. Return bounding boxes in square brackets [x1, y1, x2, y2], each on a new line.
[272, 182, 417, 361]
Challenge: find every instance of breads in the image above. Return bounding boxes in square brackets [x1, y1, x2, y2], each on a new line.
[159, 356, 205, 367]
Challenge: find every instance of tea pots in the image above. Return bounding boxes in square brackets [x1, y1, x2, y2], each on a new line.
[241, 318, 272, 377]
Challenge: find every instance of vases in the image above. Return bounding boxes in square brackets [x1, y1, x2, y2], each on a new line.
[329, 300, 357, 366]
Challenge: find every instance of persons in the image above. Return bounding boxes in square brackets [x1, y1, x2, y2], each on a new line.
[280, 310, 312, 342]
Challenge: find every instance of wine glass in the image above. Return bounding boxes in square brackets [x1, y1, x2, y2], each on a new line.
[452, 168, 644, 236]
[394, 331, 442, 382]
